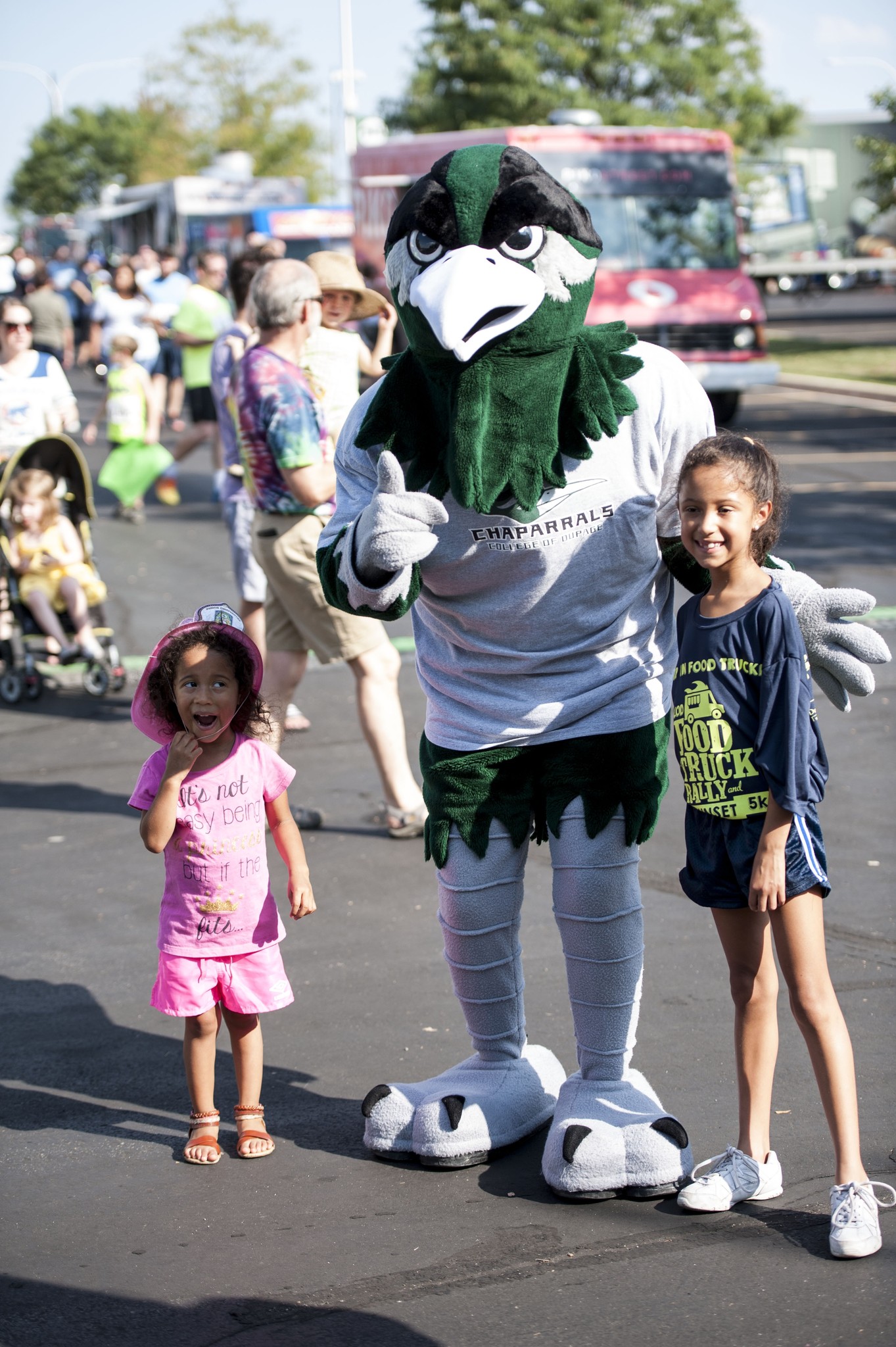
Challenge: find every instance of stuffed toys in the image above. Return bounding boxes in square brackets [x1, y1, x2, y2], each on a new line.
[315, 144, 892, 1199]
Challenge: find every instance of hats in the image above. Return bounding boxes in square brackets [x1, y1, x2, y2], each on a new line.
[302, 251, 389, 321]
[130, 602, 263, 746]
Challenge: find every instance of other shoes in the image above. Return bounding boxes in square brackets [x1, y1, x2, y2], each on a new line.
[284, 704, 311, 733]
[59, 645, 79, 660]
[156, 475, 180, 506]
[73, 636, 104, 659]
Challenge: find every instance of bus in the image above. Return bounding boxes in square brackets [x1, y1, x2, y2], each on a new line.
[16, 173, 356, 279]
[343, 105, 816, 426]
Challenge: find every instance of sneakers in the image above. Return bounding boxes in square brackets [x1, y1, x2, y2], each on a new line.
[829, 1177, 896, 1257]
[676, 1146, 783, 1211]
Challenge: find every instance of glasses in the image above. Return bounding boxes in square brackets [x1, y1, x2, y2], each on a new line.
[0, 320, 35, 333]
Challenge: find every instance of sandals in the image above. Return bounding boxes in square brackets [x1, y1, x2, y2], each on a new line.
[265, 804, 323, 832]
[386, 802, 429, 839]
[183, 1108, 223, 1164]
[233, 1104, 275, 1158]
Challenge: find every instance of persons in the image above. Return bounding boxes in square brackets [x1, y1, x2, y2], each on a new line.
[0, 216, 287, 525]
[211, 251, 430, 837]
[672, 432, 896, 1258]
[127, 621, 317, 1164]
[0, 297, 105, 661]
